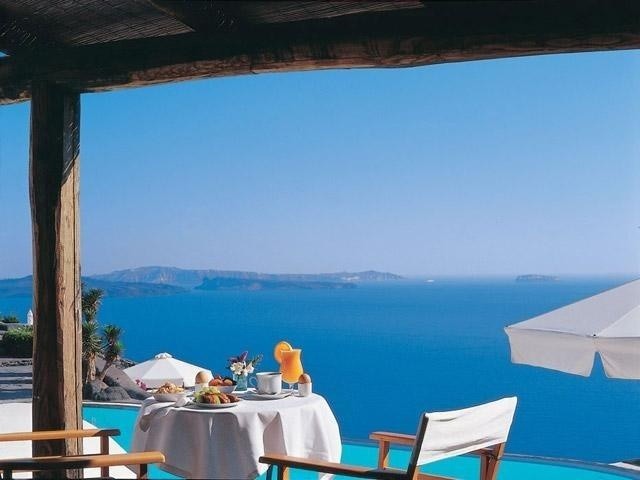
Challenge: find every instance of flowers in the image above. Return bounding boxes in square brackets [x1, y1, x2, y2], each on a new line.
[225, 351, 263, 376]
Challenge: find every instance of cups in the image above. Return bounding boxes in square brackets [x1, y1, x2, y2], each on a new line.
[297, 383, 312, 397]
[195, 383, 209, 396]
[248, 371, 283, 396]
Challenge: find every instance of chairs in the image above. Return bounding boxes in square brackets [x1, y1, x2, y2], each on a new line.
[1, 428, 166, 480]
[259, 396, 517, 480]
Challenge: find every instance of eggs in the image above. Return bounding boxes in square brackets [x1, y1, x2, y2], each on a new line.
[298, 374, 311, 383]
[196, 371, 208, 383]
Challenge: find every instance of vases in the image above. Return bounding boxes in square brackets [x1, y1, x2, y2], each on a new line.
[232, 374, 247, 391]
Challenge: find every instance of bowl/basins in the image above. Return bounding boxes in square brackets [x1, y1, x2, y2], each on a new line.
[203, 386, 237, 394]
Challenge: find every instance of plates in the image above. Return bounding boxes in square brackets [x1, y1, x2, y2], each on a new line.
[188, 399, 242, 408]
[250, 390, 293, 400]
[146, 390, 194, 402]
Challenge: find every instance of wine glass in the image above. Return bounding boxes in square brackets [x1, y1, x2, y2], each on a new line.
[279, 349, 303, 396]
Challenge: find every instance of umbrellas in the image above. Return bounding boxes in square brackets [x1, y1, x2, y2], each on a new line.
[122, 352, 214, 389]
[500, 278, 640, 382]
